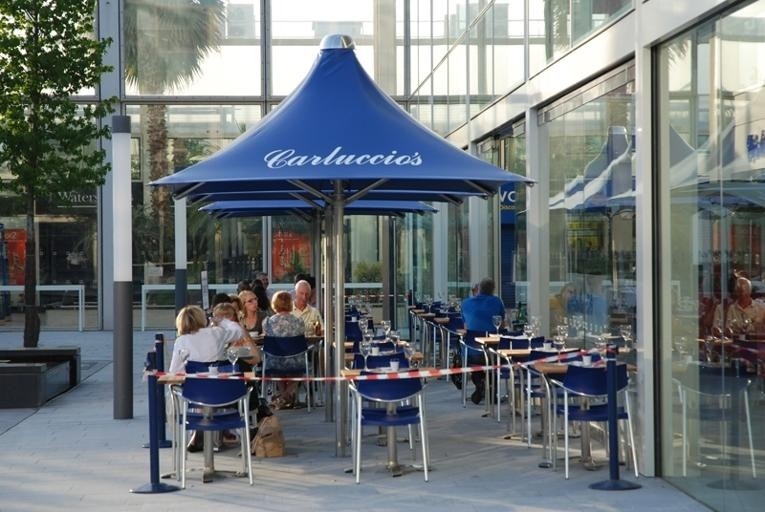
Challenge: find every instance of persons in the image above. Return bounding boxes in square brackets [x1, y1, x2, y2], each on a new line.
[547, 247, 765, 404]
[39, 243, 93, 308]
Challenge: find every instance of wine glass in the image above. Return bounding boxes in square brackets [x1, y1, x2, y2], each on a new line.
[620, 323, 632, 353]
[492, 313, 504, 338]
[522, 322, 537, 352]
[552, 333, 568, 368]
[356, 318, 395, 345]
[674, 335, 691, 368]
[356, 341, 373, 372]
[411, 290, 456, 321]
[227, 346, 241, 375]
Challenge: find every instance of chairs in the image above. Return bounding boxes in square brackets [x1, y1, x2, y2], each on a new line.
[674, 363, 759, 485]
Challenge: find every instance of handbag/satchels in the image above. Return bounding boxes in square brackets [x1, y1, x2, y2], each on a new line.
[451, 352, 462, 390]
[251, 416, 284, 457]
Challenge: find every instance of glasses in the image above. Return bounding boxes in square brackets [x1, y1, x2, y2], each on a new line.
[245, 297, 258, 302]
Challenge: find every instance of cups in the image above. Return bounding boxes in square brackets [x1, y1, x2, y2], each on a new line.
[208, 365, 219, 376]
[582, 353, 593, 367]
[543, 339, 553, 351]
[388, 356, 400, 375]
[371, 346, 380, 355]
